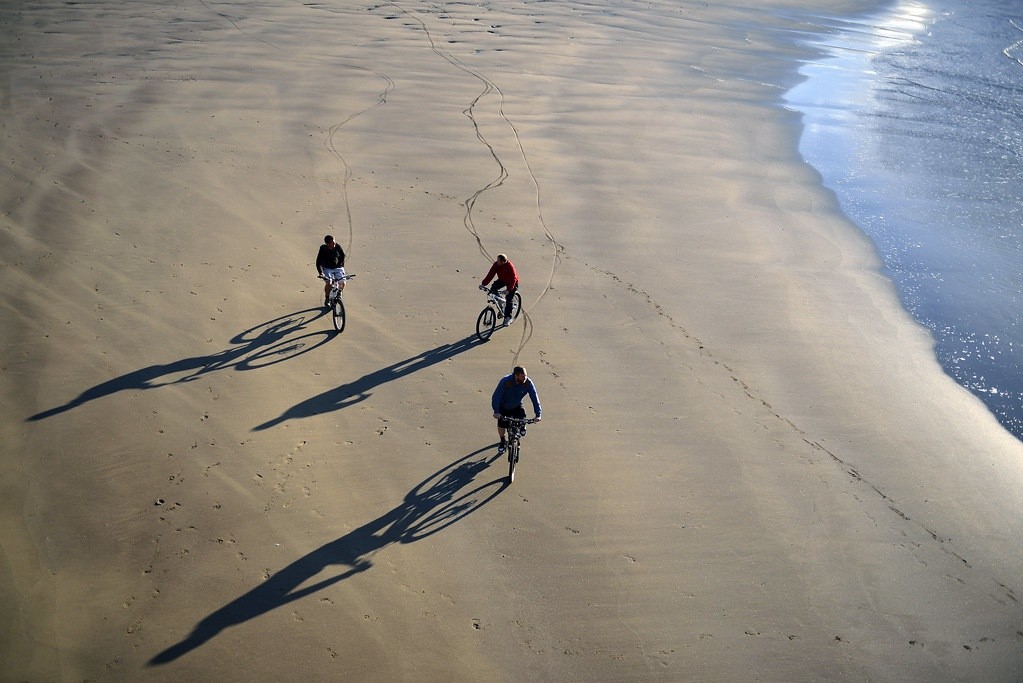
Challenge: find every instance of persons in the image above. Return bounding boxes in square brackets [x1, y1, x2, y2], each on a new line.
[492, 366, 542, 452]
[316, 234, 346, 308]
[480, 254, 519, 325]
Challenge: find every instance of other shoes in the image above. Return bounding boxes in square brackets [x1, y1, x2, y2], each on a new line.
[520, 424, 527, 436]
[324, 300, 331, 308]
[498, 440, 508, 452]
[503, 317, 514, 326]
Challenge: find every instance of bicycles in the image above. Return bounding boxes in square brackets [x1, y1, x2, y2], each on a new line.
[476, 286, 521, 341]
[493, 414, 542, 483]
[318, 274, 356, 333]
